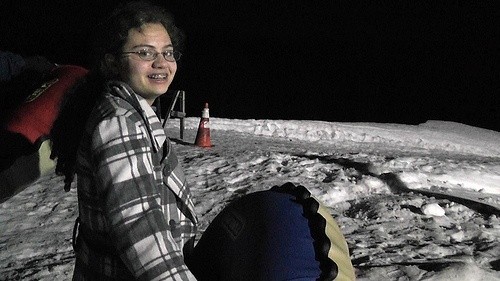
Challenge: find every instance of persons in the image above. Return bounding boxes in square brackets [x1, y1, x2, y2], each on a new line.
[70, 2, 199, 281]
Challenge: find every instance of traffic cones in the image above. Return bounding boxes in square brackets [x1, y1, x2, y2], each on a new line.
[192, 102, 216, 148]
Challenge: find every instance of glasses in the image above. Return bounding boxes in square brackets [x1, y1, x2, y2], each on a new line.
[120, 48, 182, 61]
